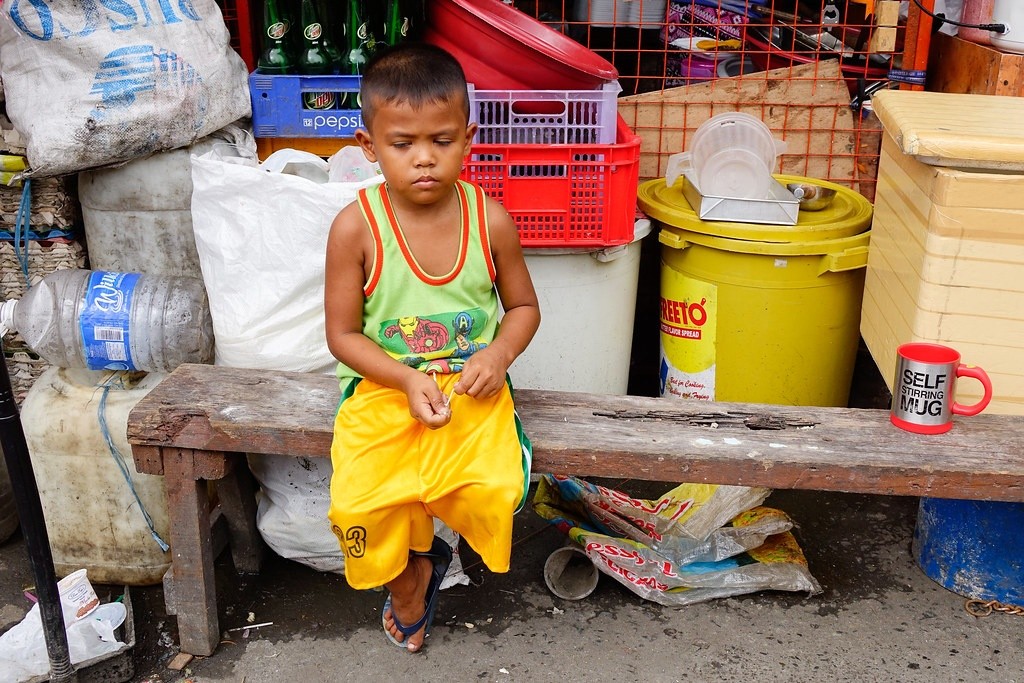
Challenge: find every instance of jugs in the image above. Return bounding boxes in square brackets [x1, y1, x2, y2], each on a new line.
[663, 112, 789, 201]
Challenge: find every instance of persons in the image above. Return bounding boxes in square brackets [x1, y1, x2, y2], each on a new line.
[324, 40, 542, 651]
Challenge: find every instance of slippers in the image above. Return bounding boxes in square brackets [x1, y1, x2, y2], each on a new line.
[381, 534, 453, 648]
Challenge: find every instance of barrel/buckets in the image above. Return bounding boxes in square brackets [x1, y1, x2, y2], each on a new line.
[73, 134, 250, 271]
[19, 364, 170, 585]
[494, 217, 651, 396]
[637, 172, 875, 408]
[909, 498, 1024, 608]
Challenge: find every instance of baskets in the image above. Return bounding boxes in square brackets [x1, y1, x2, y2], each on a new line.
[458, 80, 643, 250]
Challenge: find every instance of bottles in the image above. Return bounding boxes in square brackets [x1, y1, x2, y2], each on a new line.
[0, 267, 214, 372]
[254, 0, 414, 111]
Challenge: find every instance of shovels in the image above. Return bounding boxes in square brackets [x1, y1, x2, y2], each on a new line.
[787, 184, 837, 211]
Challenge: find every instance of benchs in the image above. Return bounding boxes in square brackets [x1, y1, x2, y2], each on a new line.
[127, 363, 1024, 656]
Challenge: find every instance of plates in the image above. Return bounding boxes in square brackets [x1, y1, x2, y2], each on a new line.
[96, 602, 127, 631]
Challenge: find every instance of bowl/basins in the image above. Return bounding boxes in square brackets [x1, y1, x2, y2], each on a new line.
[57, 568, 100, 620]
[423, 0, 621, 116]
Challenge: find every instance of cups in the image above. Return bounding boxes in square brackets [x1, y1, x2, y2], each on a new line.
[545, 540, 601, 601]
[890, 342, 991, 435]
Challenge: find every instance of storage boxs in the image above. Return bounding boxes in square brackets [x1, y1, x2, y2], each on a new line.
[248, 68, 367, 140]
[458, 80, 642, 247]
[860, 90, 1024, 416]
[680, 169, 801, 226]
